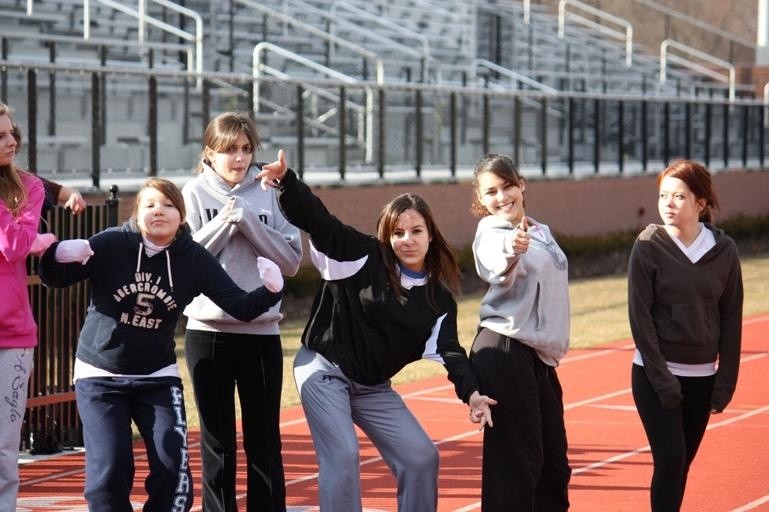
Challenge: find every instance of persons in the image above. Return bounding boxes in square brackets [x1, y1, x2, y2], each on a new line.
[255, 148, 501, 511]
[0, 100, 49, 512]
[470, 152, 575, 511]
[35, 175, 287, 510]
[174, 110, 304, 510]
[628, 157, 744, 512]
[11, 117, 89, 219]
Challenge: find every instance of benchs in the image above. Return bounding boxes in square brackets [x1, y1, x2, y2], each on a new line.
[1, 2, 768, 179]
